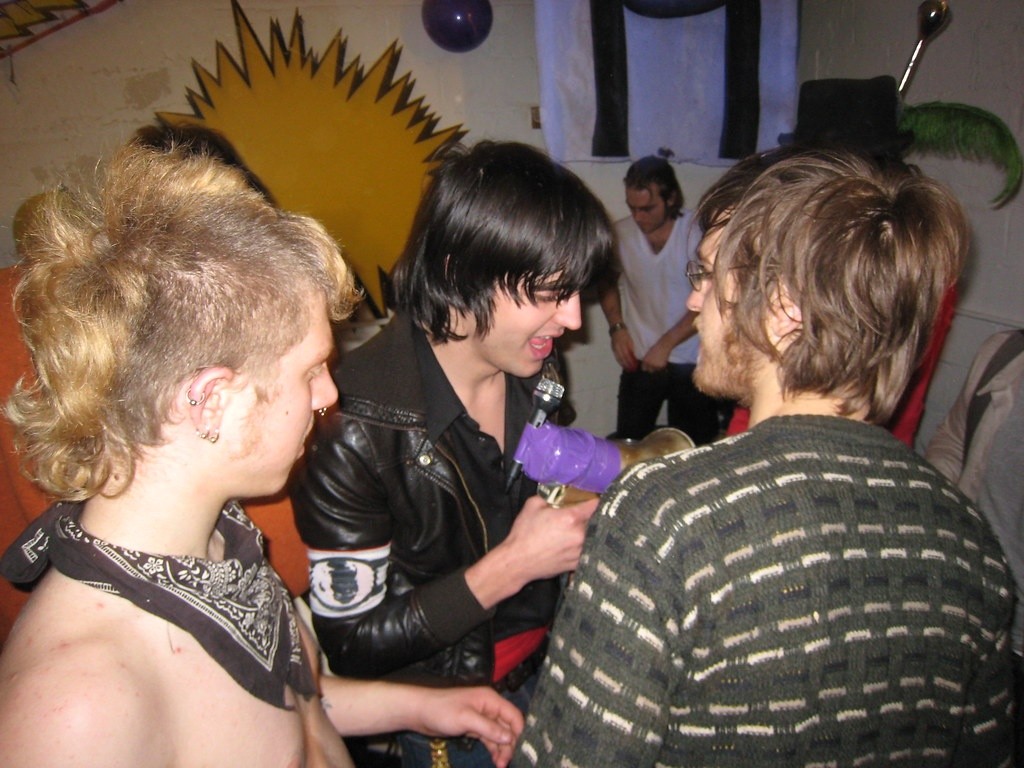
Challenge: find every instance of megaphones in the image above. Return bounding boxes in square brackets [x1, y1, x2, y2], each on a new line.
[525, 427, 696, 509]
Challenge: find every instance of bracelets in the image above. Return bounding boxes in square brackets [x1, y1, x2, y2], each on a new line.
[608, 323, 627, 336]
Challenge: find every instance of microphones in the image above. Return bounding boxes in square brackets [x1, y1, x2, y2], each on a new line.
[504, 378, 564, 494]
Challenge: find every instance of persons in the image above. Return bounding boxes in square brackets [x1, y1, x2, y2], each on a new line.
[596, 157, 719, 449]
[511, 138, 1024, 768]
[292, 143, 614, 768]
[920, 326, 1024, 696]
[0, 142, 523, 768]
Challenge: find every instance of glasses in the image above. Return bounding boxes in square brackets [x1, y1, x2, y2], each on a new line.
[685, 260, 758, 292]
